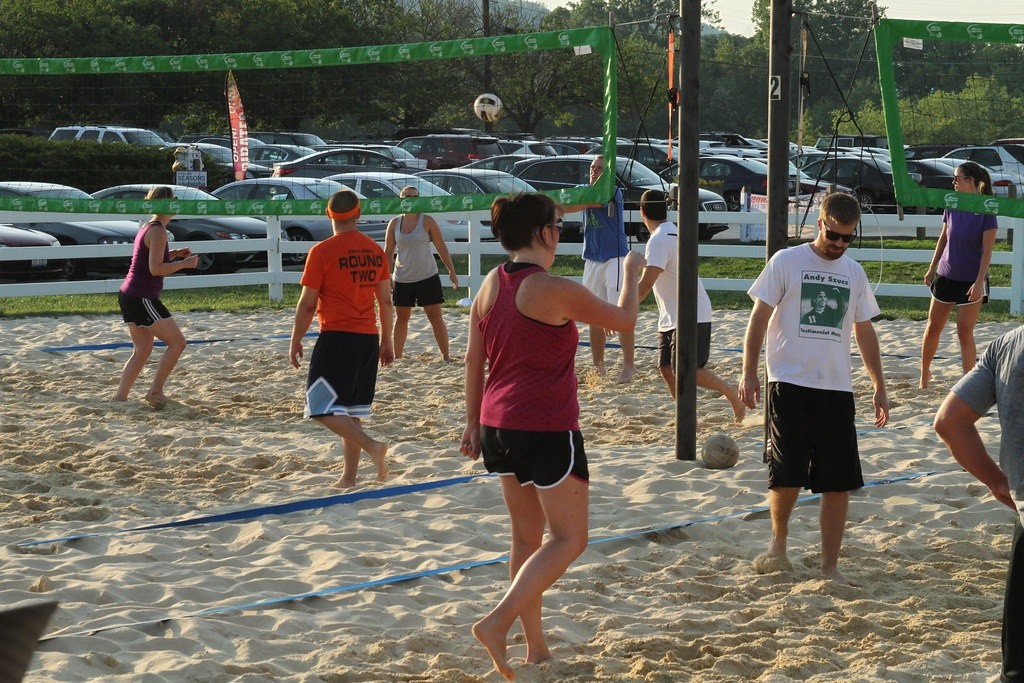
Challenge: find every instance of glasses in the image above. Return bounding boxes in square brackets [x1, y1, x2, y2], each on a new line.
[545, 217, 565, 234]
[821, 219, 858, 243]
[954, 175, 972, 182]
[590, 164, 604, 173]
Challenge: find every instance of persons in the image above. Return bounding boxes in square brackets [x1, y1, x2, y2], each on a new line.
[113, 188, 198, 404]
[289, 192, 392, 488]
[581, 156, 639, 385]
[171, 147, 188, 185]
[602, 188, 746, 426]
[932, 323, 1024, 683]
[381, 184, 458, 362]
[458, 191, 647, 681]
[919, 163, 999, 393]
[737, 192, 889, 586]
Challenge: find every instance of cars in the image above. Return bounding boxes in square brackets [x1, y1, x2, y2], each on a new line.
[273, 172, 484, 242]
[0, 224, 65, 284]
[375, 169, 585, 240]
[511, 154, 729, 244]
[164, 125, 816, 190]
[656, 153, 803, 211]
[0, 180, 175, 281]
[88, 183, 290, 276]
[703, 156, 855, 196]
[785, 132, 1024, 215]
[210, 176, 388, 266]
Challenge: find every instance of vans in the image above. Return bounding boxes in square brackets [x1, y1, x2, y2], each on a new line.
[47, 122, 170, 147]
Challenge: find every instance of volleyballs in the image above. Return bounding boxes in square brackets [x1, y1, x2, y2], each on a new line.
[474, 93, 503, 122]
[701, 434, 739, 469]
